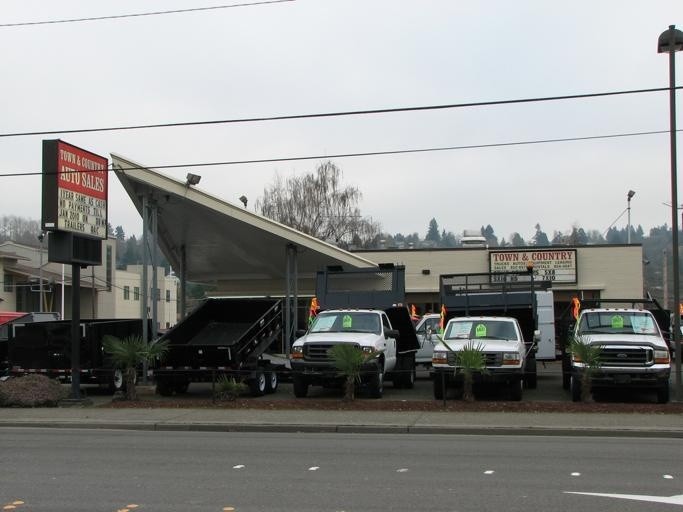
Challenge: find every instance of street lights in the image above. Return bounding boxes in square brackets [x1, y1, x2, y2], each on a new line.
[657, 25, 683, 406]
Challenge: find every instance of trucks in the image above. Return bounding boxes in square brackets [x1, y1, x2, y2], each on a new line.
[560, 292, 671, 404]
[432, 259, 539, 400]
[289, 260, 421, 399]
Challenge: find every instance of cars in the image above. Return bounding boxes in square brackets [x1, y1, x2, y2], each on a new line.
[416, 312, 443, 364]
